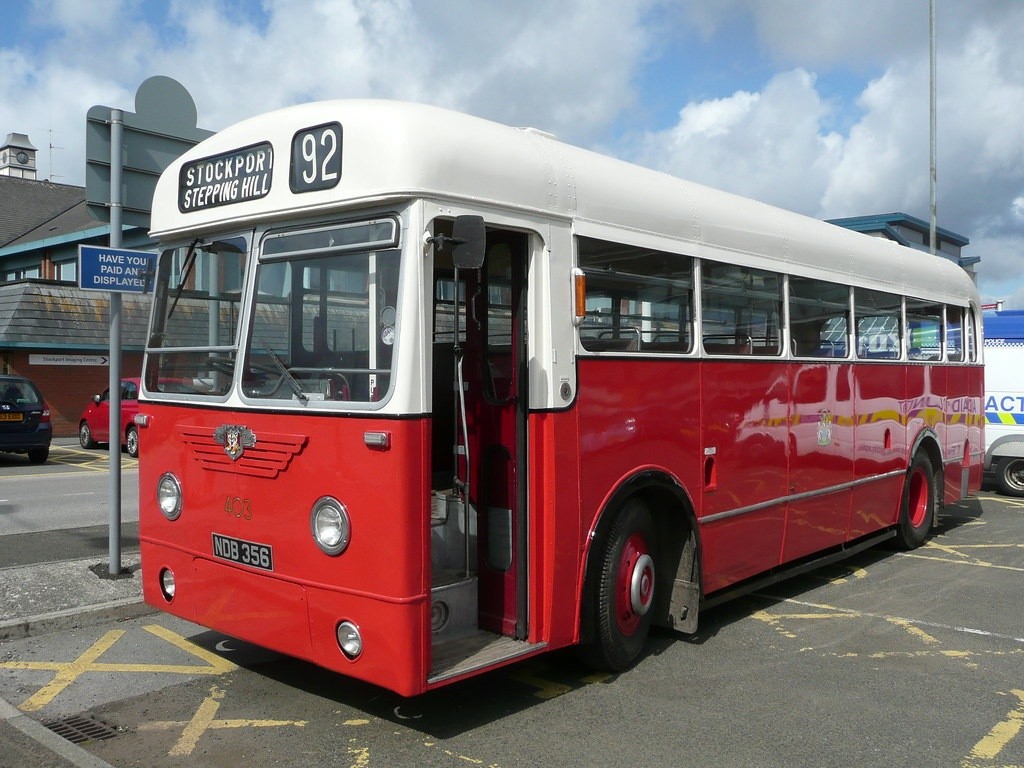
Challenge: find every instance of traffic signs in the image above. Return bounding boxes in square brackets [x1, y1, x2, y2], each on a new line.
[29, 354, 110, 366]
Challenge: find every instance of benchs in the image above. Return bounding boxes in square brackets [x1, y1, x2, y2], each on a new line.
[580, 329, 974, 362]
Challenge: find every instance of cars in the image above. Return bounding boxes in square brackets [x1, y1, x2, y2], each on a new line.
[0, 374, 53, 464]
[79, 377, 193, 459]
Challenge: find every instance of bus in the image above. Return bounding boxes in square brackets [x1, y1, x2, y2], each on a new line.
[134, 101, 985, 699]
[870, 309, 1024, 497]
